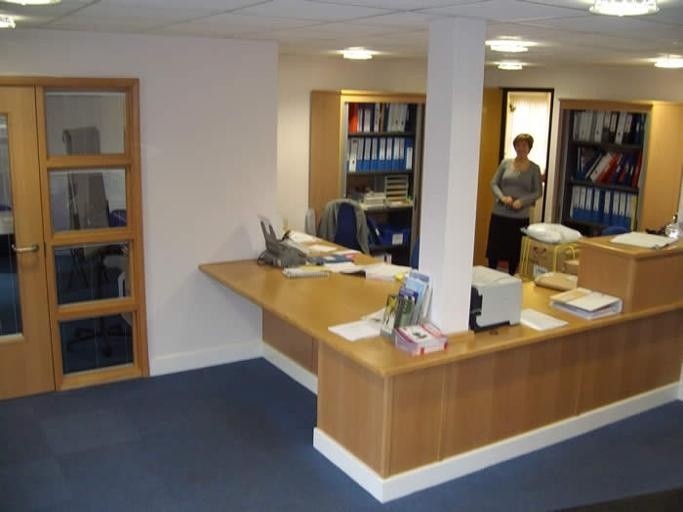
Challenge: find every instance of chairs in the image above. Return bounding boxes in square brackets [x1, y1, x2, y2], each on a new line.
[318, 197, 365, 251]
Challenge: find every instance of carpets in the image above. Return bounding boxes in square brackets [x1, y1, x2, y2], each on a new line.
[556, 485, 683, 512]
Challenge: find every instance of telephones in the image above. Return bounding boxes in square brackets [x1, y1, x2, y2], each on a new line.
[257, 220, 307, 268]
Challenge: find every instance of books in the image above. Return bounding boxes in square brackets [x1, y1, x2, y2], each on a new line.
[377, 270, 447, 355]
[608, 229, 674, 252]
[347, 183, 388, 208]
[309, 253, 352, 267]
[519, 307, 569, 336]
[547, 287, 623, 320]
[533, 269, 578, 291]
[322, 260, 413, 280]
[281, 267, 331, 280]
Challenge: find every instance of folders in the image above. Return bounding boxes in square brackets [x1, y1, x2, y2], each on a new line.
[346, 136, 415, 172]
[570, 185, 632, 228]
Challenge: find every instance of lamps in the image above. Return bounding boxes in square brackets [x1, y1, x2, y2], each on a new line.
[589, 0, 660, 15]
[489, 40, 530, 54]
[653, 54, 683, 70]
[342, 47, 372, 61]
[498, 62, 523, 70]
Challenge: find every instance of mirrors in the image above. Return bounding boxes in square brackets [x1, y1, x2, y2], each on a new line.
[500, 87, 554, 229]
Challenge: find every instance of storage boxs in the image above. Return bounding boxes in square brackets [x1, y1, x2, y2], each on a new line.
[518, 238, 575, 281]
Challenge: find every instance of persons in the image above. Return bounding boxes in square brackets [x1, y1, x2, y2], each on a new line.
[484, 133, 545, 277]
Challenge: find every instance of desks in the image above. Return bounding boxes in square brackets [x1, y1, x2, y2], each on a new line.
[199, 229, 683, 505]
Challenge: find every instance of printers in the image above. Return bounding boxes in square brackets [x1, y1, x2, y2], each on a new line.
[469, 265, 522, 332]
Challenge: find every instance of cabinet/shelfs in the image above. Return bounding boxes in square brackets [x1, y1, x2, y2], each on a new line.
[551, 97, 683, 237]
[308, 89, 426, 266]
